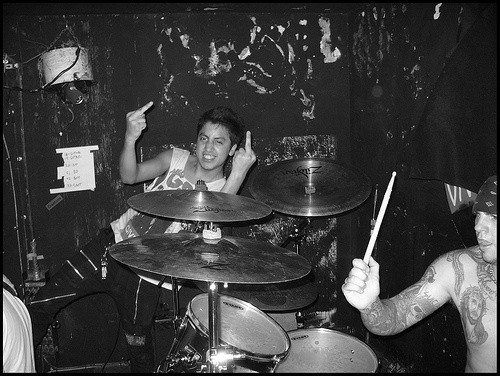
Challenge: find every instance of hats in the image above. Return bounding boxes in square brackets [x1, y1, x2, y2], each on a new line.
[472, 175, 498, 214]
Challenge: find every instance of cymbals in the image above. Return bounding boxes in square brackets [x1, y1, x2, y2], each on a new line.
[110, 231, 312, 284]
[250, 159, 372, 216]
[127, 190, 272, 223]
[196, 281, 318, 312]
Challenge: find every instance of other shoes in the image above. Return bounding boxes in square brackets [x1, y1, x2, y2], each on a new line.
[125, 331, 148, 348]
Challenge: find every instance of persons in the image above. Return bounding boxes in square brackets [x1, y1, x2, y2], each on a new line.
[341, 175, 497, 373]
[28, 101, 256, 372]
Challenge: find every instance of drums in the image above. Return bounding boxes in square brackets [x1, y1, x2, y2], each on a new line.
[166, 292, 292, 373]
[275, 327, 379, 373]
